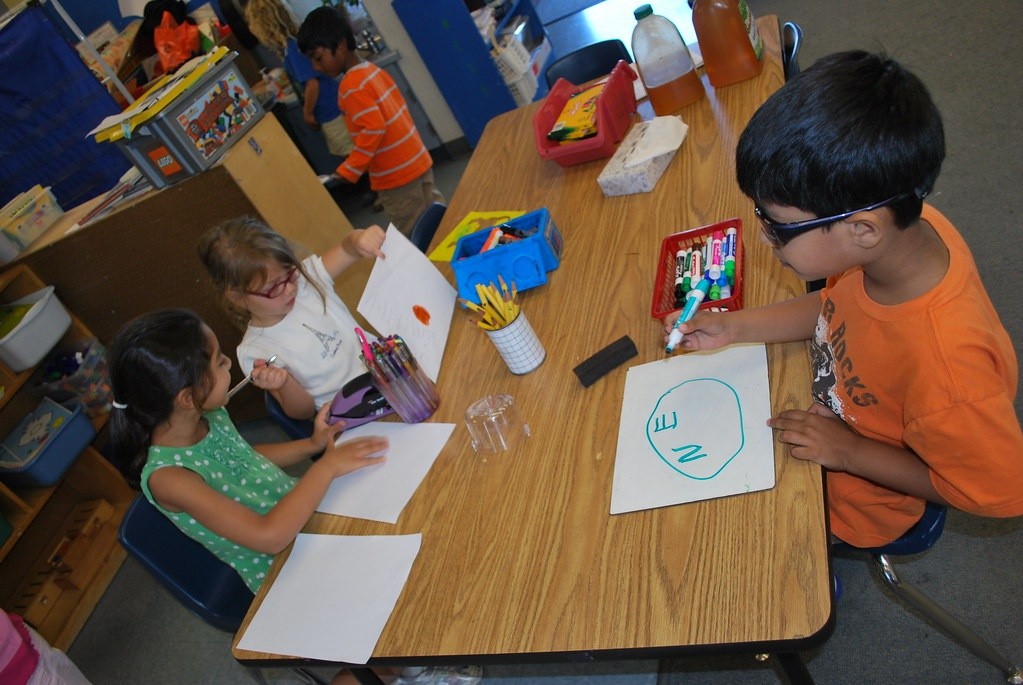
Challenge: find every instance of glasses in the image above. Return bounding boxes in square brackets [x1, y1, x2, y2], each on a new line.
[752, 192, 907, 251]
[230, 263, 300, 299]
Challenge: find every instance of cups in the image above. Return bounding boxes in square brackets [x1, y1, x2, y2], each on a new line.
[370, 357, 441, 424]
[481, 303, 547, 376]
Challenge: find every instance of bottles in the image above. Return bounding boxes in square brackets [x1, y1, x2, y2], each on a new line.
[363, 29, 379, 55]
[632, 3, 704, 117]
[692, 0, 764, 86]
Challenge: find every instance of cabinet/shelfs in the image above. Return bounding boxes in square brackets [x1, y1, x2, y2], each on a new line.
[0, 263, 139, 654]
[390, 0, 559, 151]
[277, 46, 446, 188]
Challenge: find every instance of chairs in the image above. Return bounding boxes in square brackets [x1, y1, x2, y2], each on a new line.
[783, 22, 804, 81]
[262, 387, 328, 463]
[116, 489, 320, 685]
[546, 39, 632, 89]
[826, 499, 1023, 685]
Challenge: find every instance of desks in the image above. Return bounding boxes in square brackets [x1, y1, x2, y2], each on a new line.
[0, 111, 376, 424]
[233, 18, 837, 684]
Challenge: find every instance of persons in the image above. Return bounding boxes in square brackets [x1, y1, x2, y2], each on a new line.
[109, 307, 485, 685]
[295, 7, 445, 240]
[660, 49, 1023, 662]
[194, 217, 385, 424]
[245, 0, 382, 213]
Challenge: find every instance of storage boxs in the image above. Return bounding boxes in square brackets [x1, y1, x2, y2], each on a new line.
[198, 16, 223, 52]
[0, 185, 65, 266]
[451, 209, 564, 308]
[0, 284, 73, 371]
[0, 337, 114, 488]
[93, 48, 267, 189]
[495, 15, 553, 108]
[3, 498, 114, 628]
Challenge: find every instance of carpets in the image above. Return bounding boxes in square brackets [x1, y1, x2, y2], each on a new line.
[534, 0, 606, 25]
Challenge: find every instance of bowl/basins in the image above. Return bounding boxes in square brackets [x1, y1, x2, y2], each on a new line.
[359, 38, 385, 52]
[464, 393, 532, 455]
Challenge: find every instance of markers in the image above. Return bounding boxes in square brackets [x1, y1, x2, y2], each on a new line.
[498, 237, 515, 244]
[674, 226, 735, 312]
[666, 279, 710, 352]
[500, 225, 530, 238]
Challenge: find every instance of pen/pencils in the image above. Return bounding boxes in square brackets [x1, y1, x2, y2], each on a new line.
[475, 281, 508, 326]
[228, 355, 277, 398]
[359, 335, 437, 423]
[498, 274, 521, 323]
[354, 327, 374, 361]
[456, 297, 501, 330]
